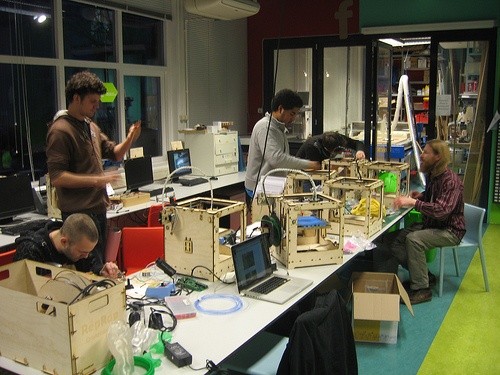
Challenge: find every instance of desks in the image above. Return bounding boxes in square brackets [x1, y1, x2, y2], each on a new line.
[0, 205, 414, 374]
[0, 166, 246, 253]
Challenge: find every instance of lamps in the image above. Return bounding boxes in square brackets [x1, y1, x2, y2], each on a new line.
[379, 37, 431, 47]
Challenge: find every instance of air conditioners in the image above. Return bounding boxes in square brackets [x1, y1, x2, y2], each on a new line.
[184, 0, 261, 21]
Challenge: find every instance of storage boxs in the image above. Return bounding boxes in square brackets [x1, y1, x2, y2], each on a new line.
[348, 272, 414, 345]
[418, 57, 427, 69]
[0, 259, 129, 375]
[413, 102, 423, 109]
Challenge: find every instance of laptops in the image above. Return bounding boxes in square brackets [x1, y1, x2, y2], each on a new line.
[230, 233, 314, 303]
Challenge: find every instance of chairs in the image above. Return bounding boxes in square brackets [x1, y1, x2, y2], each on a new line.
[437, 203, 488, 297]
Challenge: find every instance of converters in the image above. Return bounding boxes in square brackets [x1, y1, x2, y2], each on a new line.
[165, 342, 193, 367]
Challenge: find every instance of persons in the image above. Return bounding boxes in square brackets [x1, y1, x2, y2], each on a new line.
[244, 89, 320, 197]
[47, 73, 141, 264]
[296, 132, 365, 193]
[14, 215, 118, 278]
[385, 141, 466, 305]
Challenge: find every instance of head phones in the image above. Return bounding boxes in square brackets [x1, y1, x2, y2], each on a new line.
[128, 298, 177, 332]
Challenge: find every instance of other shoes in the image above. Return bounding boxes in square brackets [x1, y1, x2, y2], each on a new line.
[400, 287, 432, 305]
[401, 271, 437, 288]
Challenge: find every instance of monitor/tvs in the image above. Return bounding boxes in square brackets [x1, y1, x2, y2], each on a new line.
[167, 148, 192, 183]
[123, 155, 154, 194]
[0, 175, 35, 224]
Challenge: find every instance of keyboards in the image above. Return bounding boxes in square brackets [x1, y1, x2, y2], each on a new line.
[2, 218, 51, 235]
[148, 188, 175, 197]
[183, 178, 208, 185]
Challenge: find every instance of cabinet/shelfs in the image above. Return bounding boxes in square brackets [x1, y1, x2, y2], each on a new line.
[189, 131, 240, 177]
[376, 46, 448, 147]
[453, 41, 489, 174]
